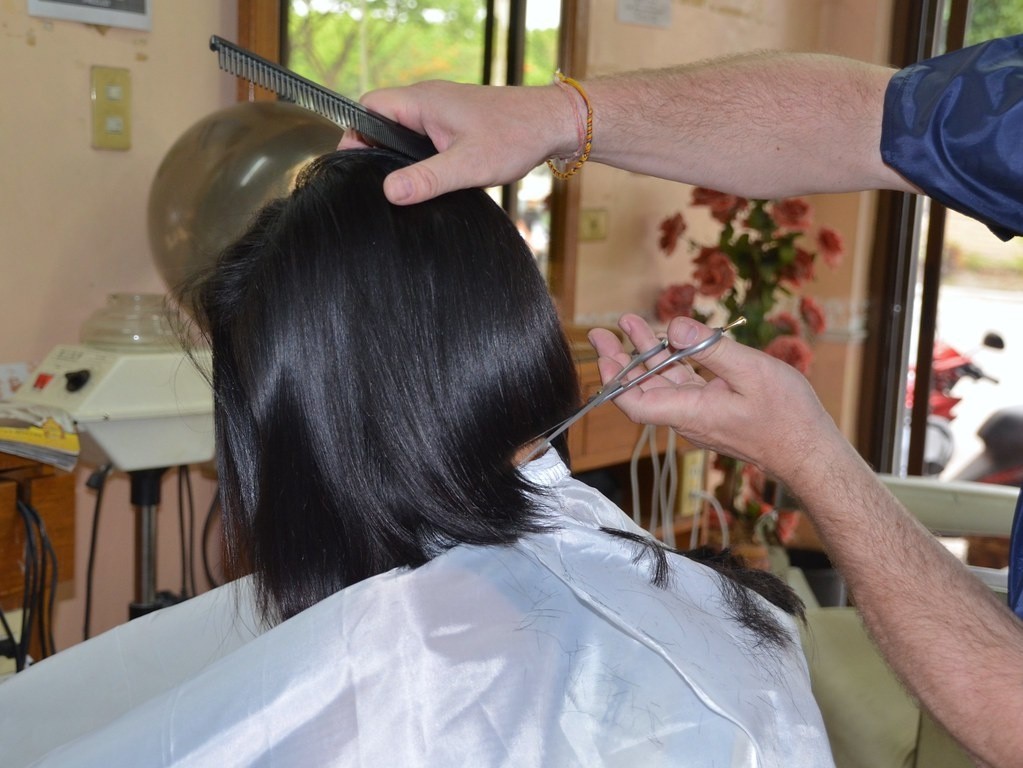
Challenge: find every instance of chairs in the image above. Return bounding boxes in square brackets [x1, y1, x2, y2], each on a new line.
[791, 481, 1021, 767]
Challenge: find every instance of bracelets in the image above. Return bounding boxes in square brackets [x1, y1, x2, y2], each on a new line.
[543, 70, 594, 182]
[551, 76, 585, 164]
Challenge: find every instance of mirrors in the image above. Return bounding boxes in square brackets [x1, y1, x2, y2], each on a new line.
[237, 0, 591, 326]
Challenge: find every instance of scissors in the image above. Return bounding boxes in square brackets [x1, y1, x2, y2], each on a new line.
[510, 316, 748, 468]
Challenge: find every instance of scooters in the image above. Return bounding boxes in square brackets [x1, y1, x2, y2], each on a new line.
[899, 332, 1005, 475]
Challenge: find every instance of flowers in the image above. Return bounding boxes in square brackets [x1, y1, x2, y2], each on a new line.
[655, 179, 842, 571]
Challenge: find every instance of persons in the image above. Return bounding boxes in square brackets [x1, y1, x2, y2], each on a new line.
[338, 21, 1021, 768]
[0, 142, 839, 767]
